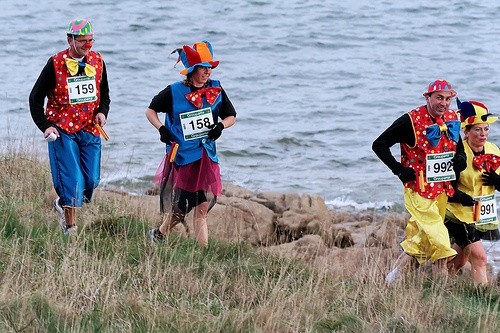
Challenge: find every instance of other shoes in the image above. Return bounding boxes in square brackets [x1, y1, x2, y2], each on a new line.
[147, 229, 166, 247]
[52, 197, 67, 230]
[62, 225, 78, 237]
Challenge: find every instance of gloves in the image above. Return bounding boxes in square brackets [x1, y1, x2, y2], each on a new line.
[450, 156, 467, 173]
[481, 167, 500, 191]
[459, 193, 474, 206]
[397, 167, 416, 182]
[208, 122, 225, 140]
[159, 126, 174, 145]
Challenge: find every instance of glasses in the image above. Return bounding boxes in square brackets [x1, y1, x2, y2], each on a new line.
[74, 39, 95, 45]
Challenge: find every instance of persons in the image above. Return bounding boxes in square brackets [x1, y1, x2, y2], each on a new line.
[28, 18, 110, 237]
[444, 97, 500, 283]
[145, 41, 236, 250]
[371, 79, 467, 296]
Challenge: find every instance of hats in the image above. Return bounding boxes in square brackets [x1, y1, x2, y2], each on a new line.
[171, 40, 219, 74]
[65, 19, 95, 36]
[422, 79, 457, 99]
[455, 96, 499, 129]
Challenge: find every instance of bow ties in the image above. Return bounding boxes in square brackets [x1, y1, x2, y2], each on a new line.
[472, 153, 500, 172]
[426, 119, 461, 147]
